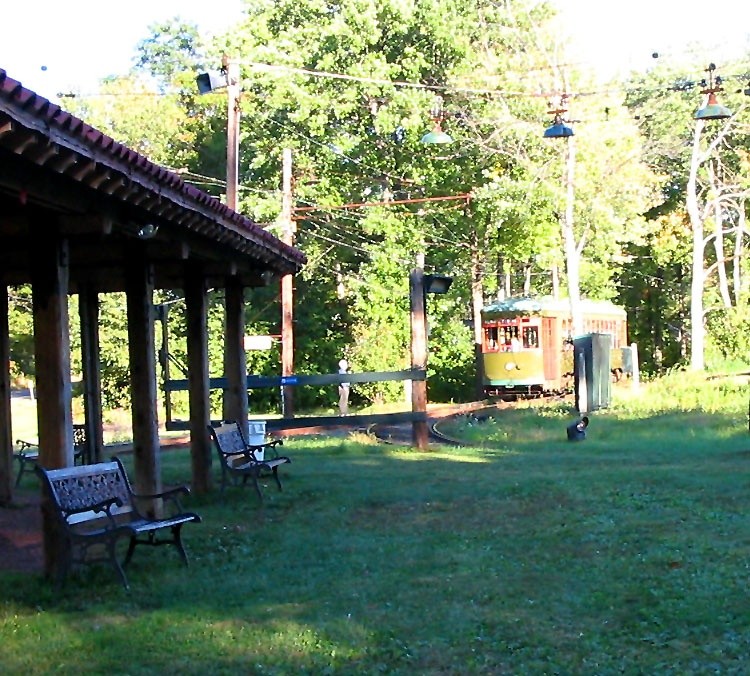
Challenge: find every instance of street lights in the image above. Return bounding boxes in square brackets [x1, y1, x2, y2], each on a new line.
[191, 59, 240, 210]
[408, 268, 456, 452]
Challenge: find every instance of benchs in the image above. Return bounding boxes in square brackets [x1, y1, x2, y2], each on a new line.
[34, 455, 204, 593]
[13, 423, 85, 488]
[210, 418, 291, 498]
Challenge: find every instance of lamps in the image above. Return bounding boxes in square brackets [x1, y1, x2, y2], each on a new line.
[421, 121, 453, 145]
[692, 92, 734, 121]
[543, 113, 576, 138]
[135, 217, 161, 240]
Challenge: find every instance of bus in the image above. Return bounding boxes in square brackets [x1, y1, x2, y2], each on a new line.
[482, 291, 628, 396]
